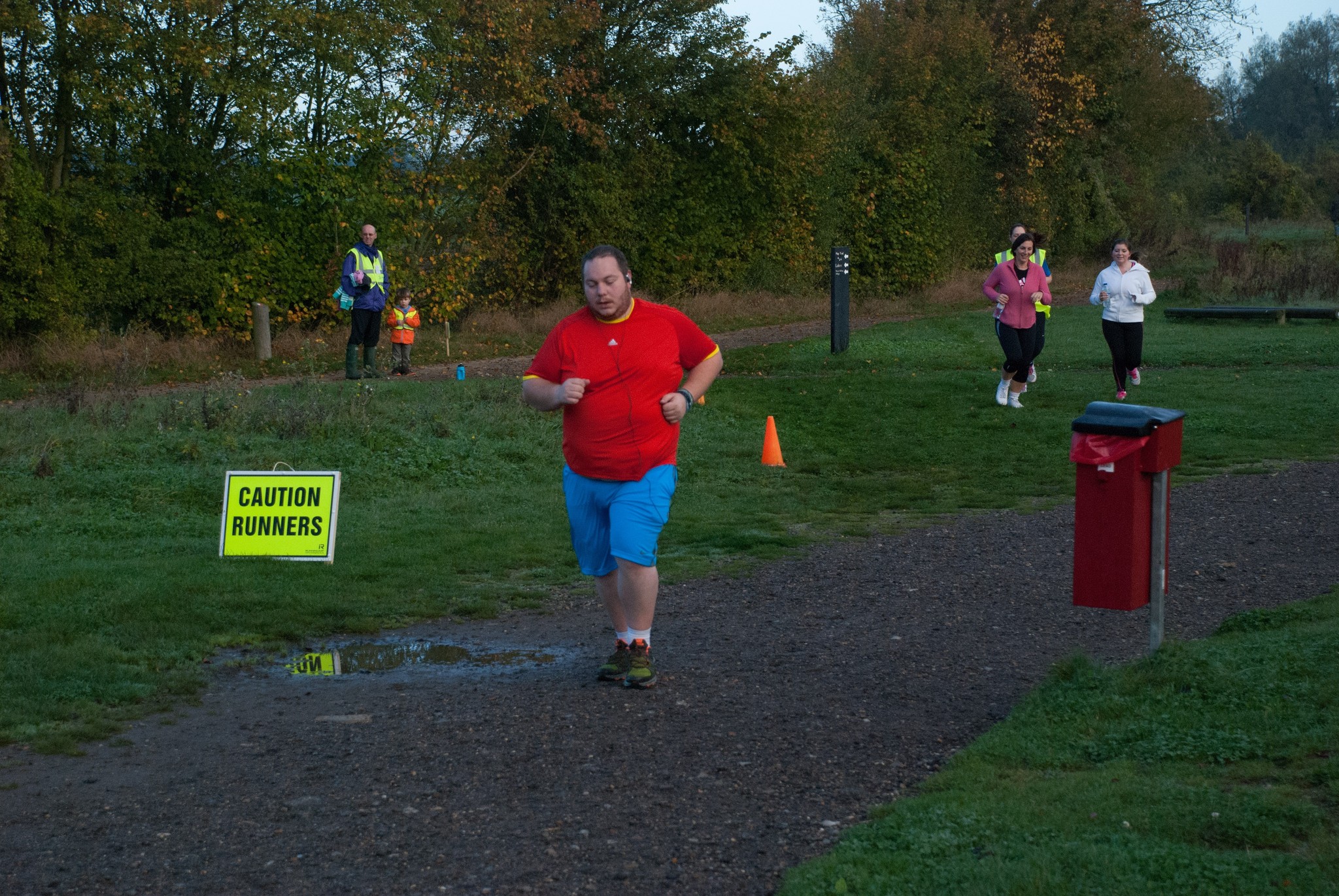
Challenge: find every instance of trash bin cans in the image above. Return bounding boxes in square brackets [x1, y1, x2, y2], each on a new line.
[1071, 400, 1186, 612]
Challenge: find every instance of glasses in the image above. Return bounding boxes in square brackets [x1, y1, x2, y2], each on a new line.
[362, 232, 376, 238]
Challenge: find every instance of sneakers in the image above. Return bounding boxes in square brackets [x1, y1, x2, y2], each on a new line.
[624, 638, 658, 688]
[1007, 397, 1025, 409]
[401, 367, 414, 375]
[391, 368, 401, 376]
[1116, 390, 1126, 400]
[1026, 361, 1037, 382]
[1126, 366, 1140, 386]
[996, 382, 1009, 406]
[1020, 381, 1027, 393]
[596, 638, 630, 680]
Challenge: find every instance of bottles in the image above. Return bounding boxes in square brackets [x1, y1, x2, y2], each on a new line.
[456, 363, 465, 380]
[993, 302, 1005, 319]
[1102, 283, 1111, 307]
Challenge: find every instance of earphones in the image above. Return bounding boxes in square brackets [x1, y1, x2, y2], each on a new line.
[627, 275, 632, 284]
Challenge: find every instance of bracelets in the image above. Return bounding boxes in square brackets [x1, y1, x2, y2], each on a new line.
[676, 387, 694, 411]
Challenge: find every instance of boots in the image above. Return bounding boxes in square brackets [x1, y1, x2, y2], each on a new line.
[346, 343, 366, 379]
[362, 346, 387, 378]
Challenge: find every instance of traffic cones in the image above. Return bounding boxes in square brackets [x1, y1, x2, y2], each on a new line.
[761, 416, 787, 469]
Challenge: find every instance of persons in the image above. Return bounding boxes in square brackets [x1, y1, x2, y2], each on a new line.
[1089, 238, 1157, 401]
[982, 224, 1053, 408]
[341, 224, 390, 380]
[387, 288, 420, 375]
[521, 244, 724, 689]
